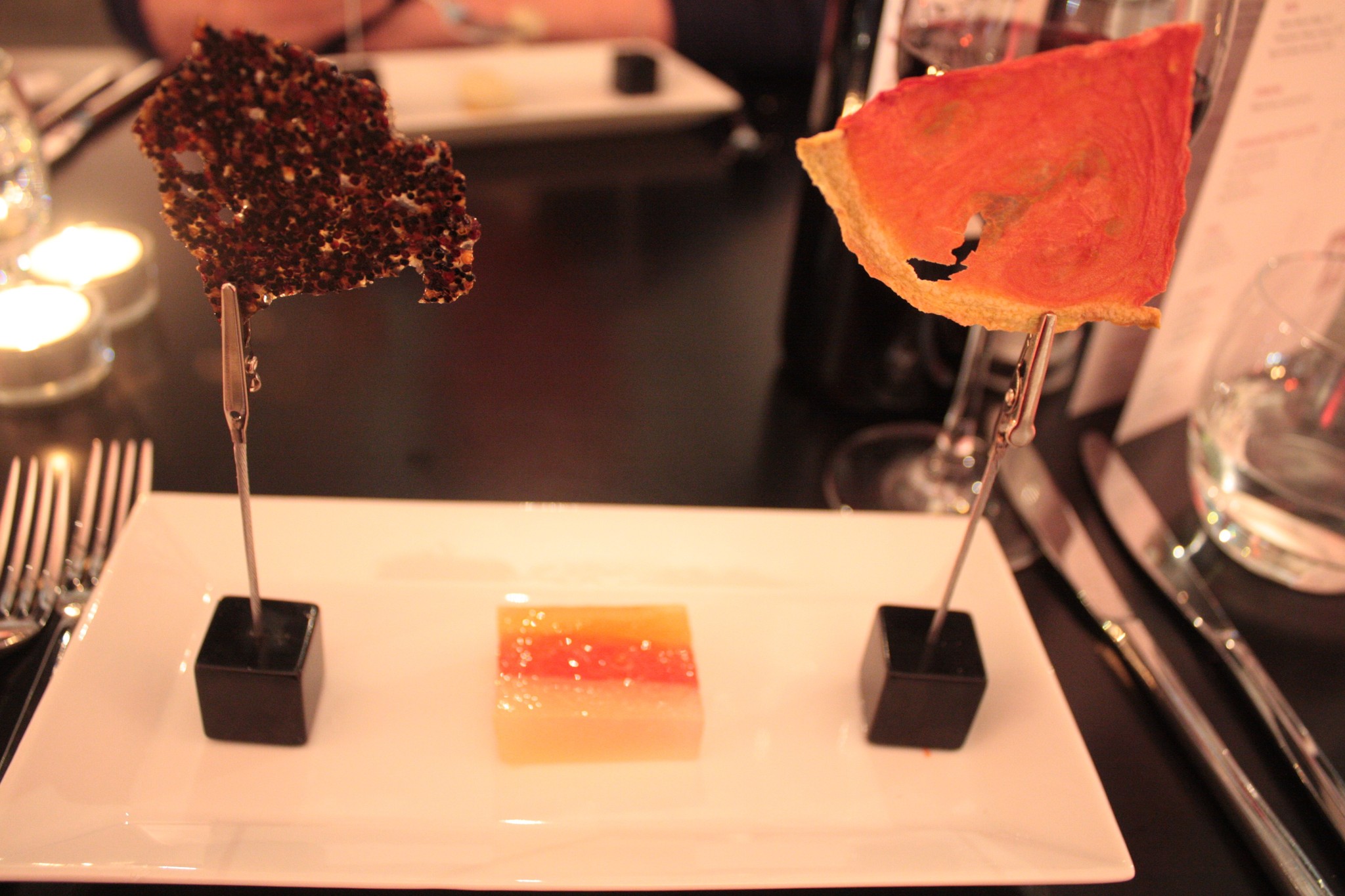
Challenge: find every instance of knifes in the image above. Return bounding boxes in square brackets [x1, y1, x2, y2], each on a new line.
[1078, 428, 1344, 840]
[987, 404, 1344, 896]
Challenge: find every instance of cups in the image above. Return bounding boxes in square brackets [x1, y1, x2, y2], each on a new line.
[1, 286, 116, 409]
[0, 46, 52, 279]
[1185, 252, 1343, 599]
[22, 224, 173, 332]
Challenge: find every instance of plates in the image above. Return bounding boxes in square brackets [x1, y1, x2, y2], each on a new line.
[1, 489, 1136, 891]
[319, 34, 748, 151]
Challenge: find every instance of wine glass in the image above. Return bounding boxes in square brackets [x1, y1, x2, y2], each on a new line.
[824, 0, 1191, 571]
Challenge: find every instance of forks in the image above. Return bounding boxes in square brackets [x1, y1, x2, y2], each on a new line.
[0, 438, 156, 782]
[0, 453, 70, 647]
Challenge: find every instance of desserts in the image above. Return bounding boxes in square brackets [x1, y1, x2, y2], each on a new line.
[492, 602, 706, 762]
